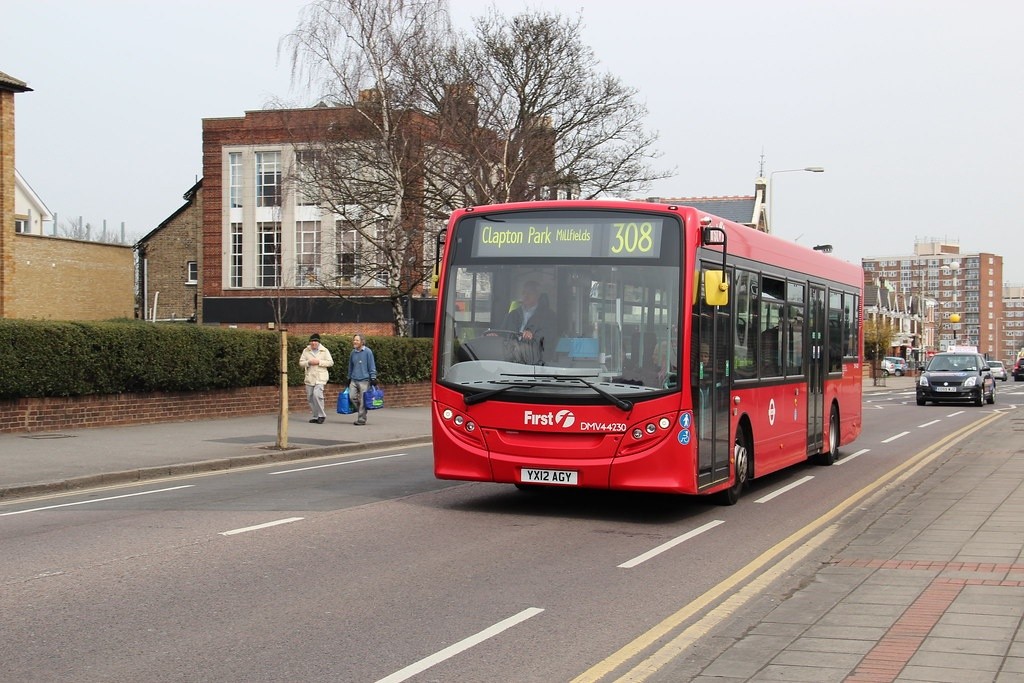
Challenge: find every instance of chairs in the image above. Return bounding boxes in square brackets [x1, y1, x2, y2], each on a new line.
[557, 312, 826, 374]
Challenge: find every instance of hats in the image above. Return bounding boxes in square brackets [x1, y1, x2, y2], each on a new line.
[309, 334, 320, 342]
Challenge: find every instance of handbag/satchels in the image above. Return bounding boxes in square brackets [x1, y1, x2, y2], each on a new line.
[336, 387, 358, 414]
[363, 385, 384, 410]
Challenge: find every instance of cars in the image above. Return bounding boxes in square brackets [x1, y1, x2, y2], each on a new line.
[916, 352, 997, 407]
[985, 361, 1008, 382]
[881, 360, 895, 377]
[885, 357, 908, 377]
[1013, 358, 1024, 381]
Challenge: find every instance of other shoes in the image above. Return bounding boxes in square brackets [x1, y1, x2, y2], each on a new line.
[309, 419, 318, 423]
[354, 421, 365, 425]
[317, 417, 325, 423]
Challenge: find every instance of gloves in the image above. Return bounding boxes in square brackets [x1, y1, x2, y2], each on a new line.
[370, 379, 378, 386]
[345, 380, 351, 387]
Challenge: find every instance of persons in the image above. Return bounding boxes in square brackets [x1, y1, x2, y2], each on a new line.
[966, 361, 974, 368]
[701, 343, 709, 367]
[346, 334, 378, 425]
[485, 281, 553, 352]
[299, 333, 334, 424]
[653, 325, 678, 371]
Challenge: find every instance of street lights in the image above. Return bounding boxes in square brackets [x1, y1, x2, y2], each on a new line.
[768, 167, 824, 236]
[940, 262, 967, 346]
[996, 317, 1003, 363]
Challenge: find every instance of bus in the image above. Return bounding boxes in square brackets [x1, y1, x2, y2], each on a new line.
[431, 199, 863, 506]
